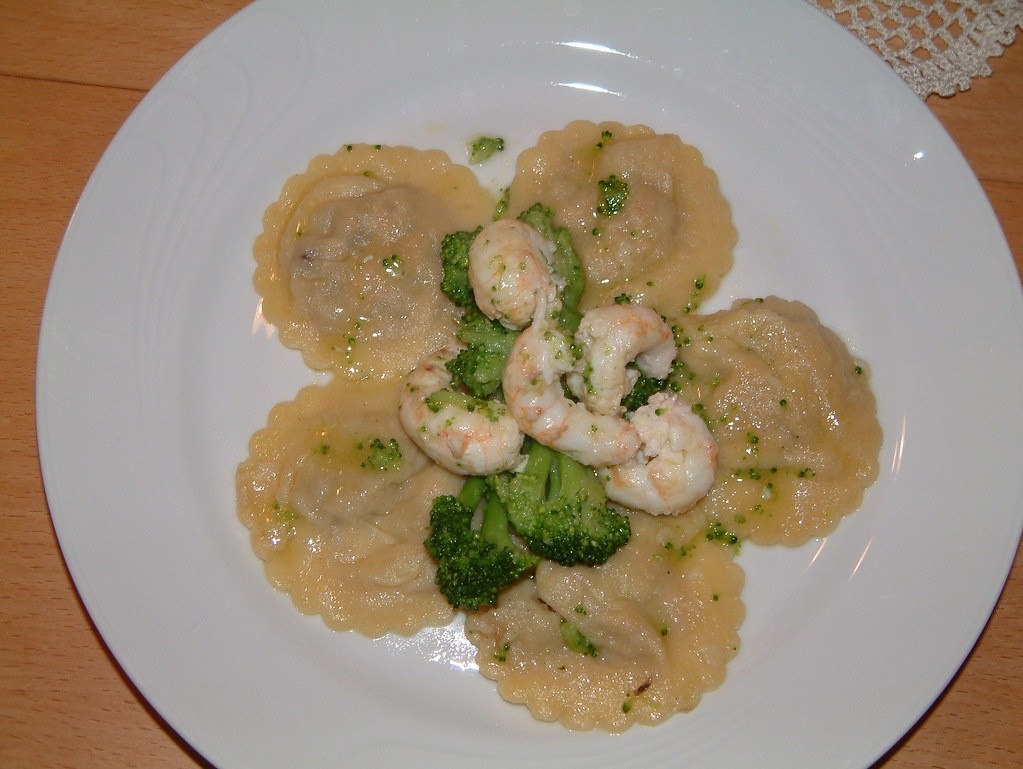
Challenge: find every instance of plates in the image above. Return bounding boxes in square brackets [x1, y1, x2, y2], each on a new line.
[37, 0, 1023, 769]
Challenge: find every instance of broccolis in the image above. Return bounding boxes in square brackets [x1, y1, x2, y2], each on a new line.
[427, 205, 669, 610]
